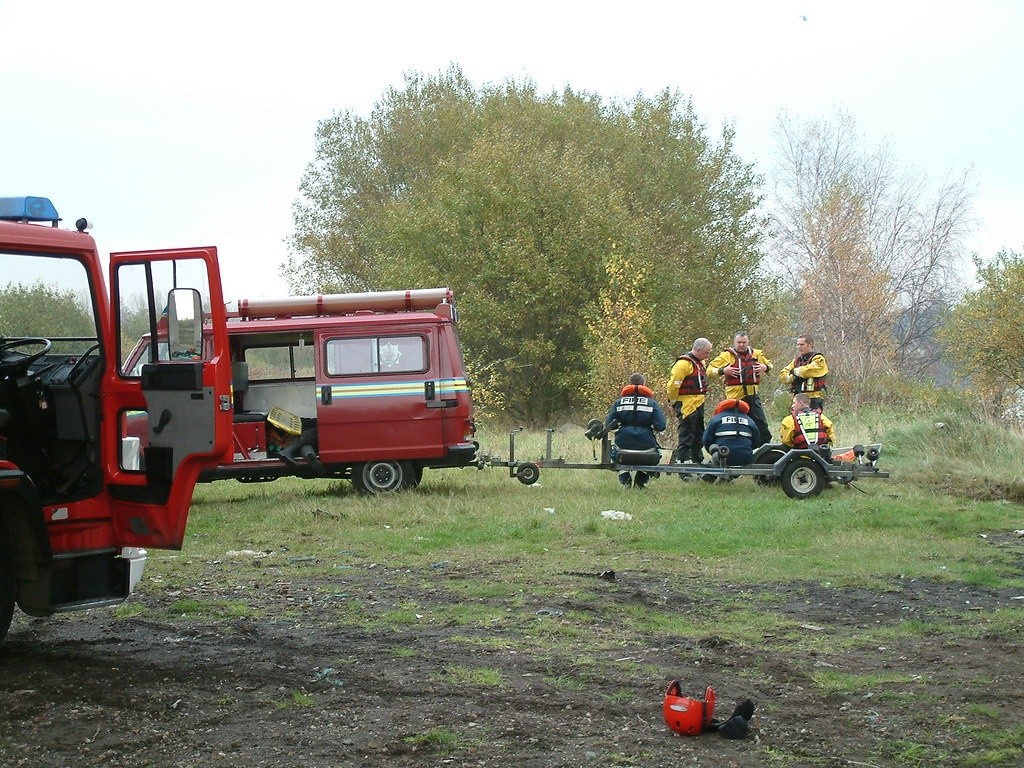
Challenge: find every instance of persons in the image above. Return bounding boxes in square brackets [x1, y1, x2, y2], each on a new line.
[706, 331, 774, 447]
[779, 334, 829, 414]
[780, 393, 836, 488]
[277, 418, 325, 477]
[702, 399, 760, 486]
[604, 372, 667, 494]
[666, 338, 713, 482]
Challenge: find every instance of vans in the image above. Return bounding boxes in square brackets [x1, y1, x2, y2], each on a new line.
[115, 286, 479, 500]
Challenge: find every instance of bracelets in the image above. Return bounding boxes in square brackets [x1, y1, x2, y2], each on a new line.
[764, 365, 771, 372]
[718, 367, 724, 376]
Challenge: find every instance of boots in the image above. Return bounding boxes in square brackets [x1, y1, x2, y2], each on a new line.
[279, 431, 309, 470]
[299, 443, 327, 475]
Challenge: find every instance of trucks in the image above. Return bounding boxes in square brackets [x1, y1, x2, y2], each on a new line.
[0, 193, 237, 652]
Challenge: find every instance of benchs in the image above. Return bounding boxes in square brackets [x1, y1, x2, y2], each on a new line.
[233, 410, 269, 451]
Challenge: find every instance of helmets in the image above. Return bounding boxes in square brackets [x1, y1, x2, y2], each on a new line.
[663, 679, 715, 737]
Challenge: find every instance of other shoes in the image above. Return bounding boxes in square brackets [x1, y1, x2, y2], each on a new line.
[616, 469, 632, 488]
[633, 466, 650, 489]
